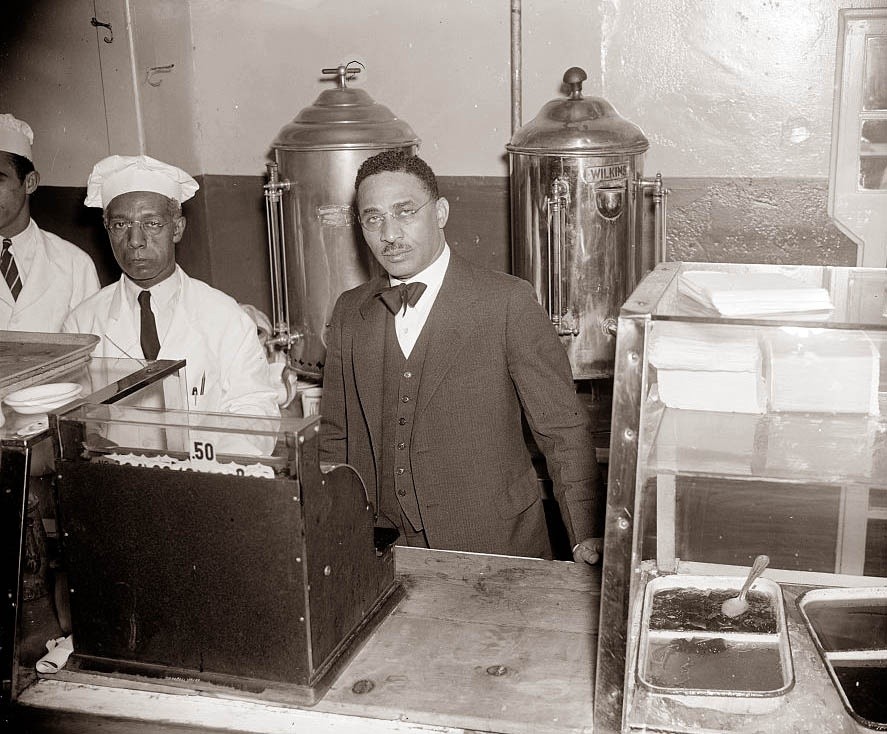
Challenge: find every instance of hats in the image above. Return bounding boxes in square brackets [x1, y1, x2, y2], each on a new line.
[84, 155, 199, 212]
[0, 113, 34, 164]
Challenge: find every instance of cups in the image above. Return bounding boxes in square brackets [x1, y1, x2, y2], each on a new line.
[302, 388, 326, 431]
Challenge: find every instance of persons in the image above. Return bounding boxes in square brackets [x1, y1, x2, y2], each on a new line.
[61, 155, 280, 457]
[0, 113, 100, 334]
[316, 151, 605, 565]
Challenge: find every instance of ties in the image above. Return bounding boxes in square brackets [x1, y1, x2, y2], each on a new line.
[0, 239, 24, 303]
[138, 290, 161, 361]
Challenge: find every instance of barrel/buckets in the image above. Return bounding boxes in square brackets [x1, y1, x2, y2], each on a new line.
[505, 67, 666, 380]
[263, 66, 420, 378]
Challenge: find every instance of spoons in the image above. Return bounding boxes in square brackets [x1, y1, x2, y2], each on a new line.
[721, 555, 770, 618]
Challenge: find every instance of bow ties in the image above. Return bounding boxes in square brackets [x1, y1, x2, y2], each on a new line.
[358, 282, 427, 320]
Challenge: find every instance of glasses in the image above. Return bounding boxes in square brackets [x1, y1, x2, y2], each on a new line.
[357, 198, 434, 232]
[106, 217, 181, 237]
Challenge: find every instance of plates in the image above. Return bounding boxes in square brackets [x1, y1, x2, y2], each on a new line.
[3, 383, 82, 414]
[637, 574, 795, 715]
[797, 587, 887, 731]
